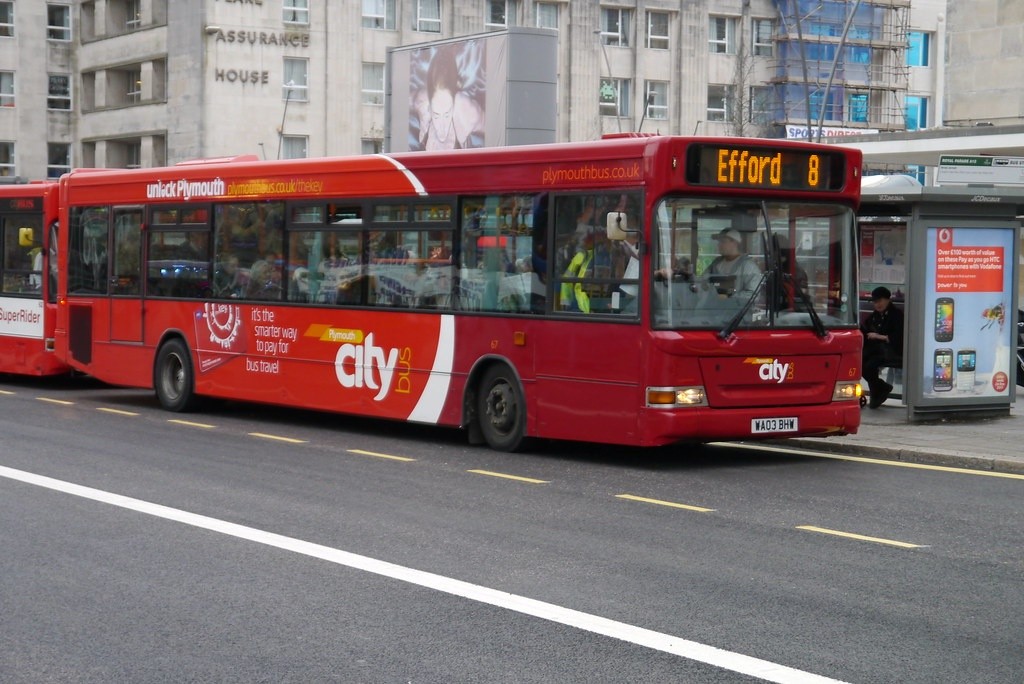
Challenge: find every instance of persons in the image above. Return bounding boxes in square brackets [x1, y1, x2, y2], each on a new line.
[859, 285, 903, 407]
[409, 48, 486, 151]
[561, 229, 671, 312]
[700, 228, 808, 320]
[216, 239, 531, 310]
[27, 231, 210, 298]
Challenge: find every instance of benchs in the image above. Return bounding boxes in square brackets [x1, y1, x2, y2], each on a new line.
[864, 361, 902, 399]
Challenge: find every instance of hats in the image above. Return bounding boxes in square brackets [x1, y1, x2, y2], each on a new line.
[868, 286, 891, 303]
[712, 228, 742, 244]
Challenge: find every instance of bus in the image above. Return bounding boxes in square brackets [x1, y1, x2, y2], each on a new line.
[0, 178, 208, 376]
[52, 132, 864, 454]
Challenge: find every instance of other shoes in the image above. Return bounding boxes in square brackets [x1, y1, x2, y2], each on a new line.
[869, 379, 893, 409]
